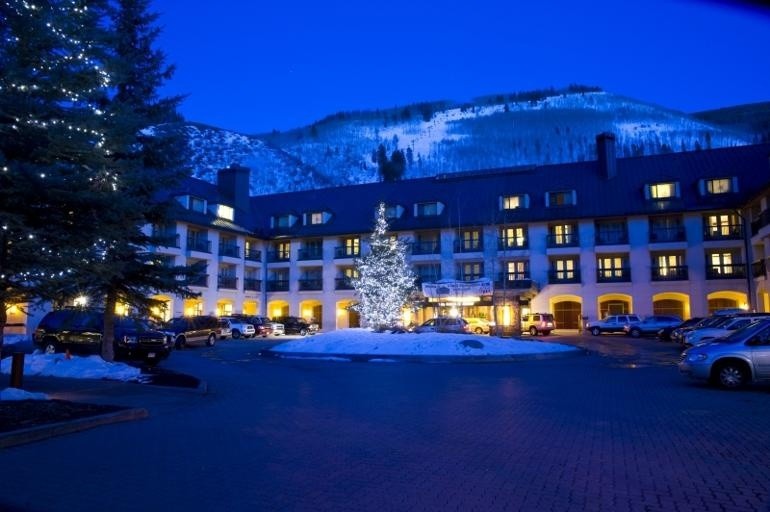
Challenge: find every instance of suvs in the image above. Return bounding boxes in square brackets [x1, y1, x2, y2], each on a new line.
[33, 304, 321, 371]
[520, 311, 555, 336]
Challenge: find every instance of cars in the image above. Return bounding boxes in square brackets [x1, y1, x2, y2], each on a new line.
[585, 311, 770, 388]
[461, 318, 496, 334]
[415, 316, 471, 333]
